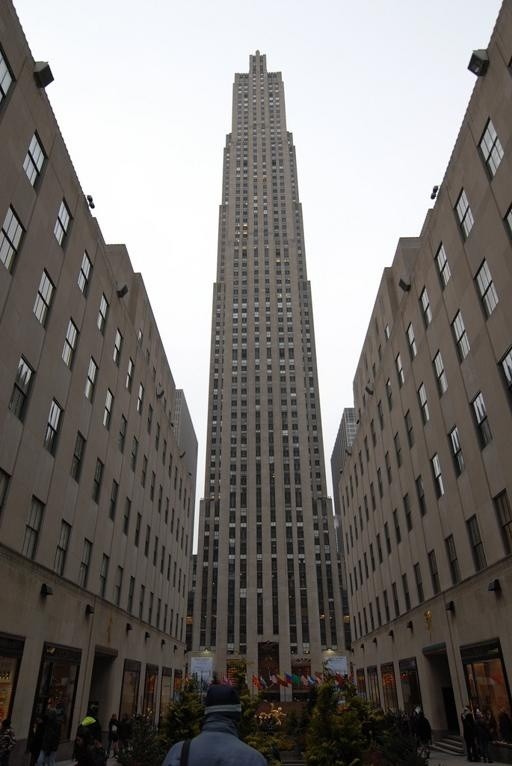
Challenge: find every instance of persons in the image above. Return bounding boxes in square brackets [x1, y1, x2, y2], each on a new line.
[107, 712, 132, 759]
[73, 702, 107, 766]
[461, 706, 498, 762]
[162, 684, 268, 766]
[28, 699, 60, 764]
[0, 718, 17, 766]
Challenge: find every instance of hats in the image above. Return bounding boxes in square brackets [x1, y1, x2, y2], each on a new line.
[205, 684, 242, 715]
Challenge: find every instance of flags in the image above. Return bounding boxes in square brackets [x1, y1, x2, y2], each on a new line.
[175, 672, 355, 690]
